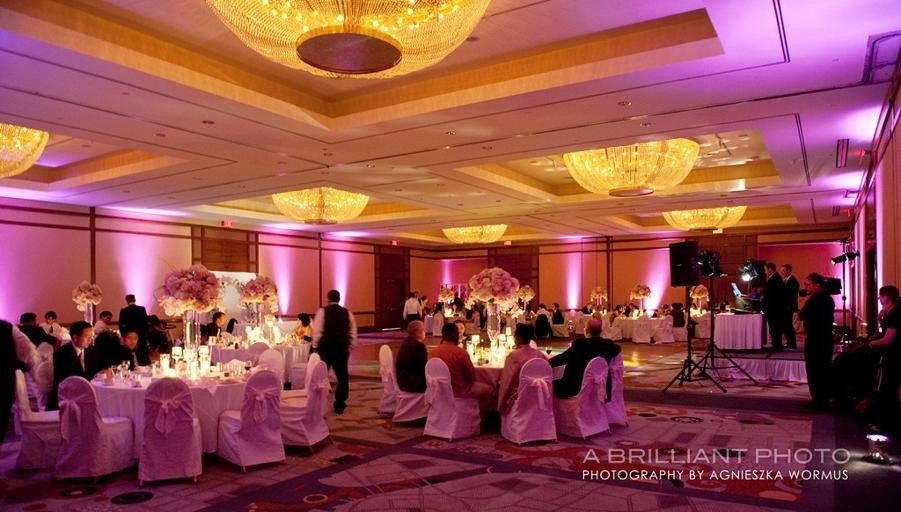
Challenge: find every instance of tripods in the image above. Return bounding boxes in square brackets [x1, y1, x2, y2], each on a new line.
[659, 286, 763, 393]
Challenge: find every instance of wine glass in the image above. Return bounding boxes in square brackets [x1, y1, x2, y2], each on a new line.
[209, 326, 305, 348]
[465, 327, 514, 365]
[95, 345, 252, 388]
[545, 346, 552, 357]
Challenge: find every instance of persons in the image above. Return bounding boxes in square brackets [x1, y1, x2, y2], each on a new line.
[799, 273, 834, 413]
[0, 295, 240, 443]
[868, 285, 901, 439]
[290, 313, 315, 354]
[742, 262, 801, 352]
[312, 290, 358, 414]
[395, 290, 685, 417]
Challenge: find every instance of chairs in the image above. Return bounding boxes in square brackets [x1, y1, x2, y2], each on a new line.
[420, 355, 483, 441]
[422, 305, 712, 346]
[553, 356, 611, 443]
[603, 353, 630, 430]
[0, 313, 338, 490]
[378, 343, 426, 426]
[499, 358, 559, 447]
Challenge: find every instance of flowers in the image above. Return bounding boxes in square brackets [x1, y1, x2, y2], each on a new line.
[237, 277, 280, 314]
[435, 266, 536, 305]
[630, 282, 651, 301]
[153, 258, 221, 316]
[589, 286, 609, 303]
[72, 280, 103, 312]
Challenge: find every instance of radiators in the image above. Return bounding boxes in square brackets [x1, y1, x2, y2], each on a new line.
[689, 284, 709, 303]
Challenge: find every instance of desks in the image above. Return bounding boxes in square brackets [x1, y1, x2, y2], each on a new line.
[714, 312, 763, 352]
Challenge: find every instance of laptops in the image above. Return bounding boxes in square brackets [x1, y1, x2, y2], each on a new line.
[731, 282, 748, 296]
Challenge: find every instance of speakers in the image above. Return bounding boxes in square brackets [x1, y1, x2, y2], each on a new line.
[700, 251, 719, 277]
[669, 240, 698, 286]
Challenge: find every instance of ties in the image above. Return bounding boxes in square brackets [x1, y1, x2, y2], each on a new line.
[79, 352, 83, 370]
[50, 326, 53, 334]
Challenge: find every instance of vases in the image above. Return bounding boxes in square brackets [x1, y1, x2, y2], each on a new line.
[253, 303, 266, 332]
[595, 299, 603, 308]
[182, 310, 200, 366]
[82, 301, 93, 324]
[695, 298, 701, 312]
[638, 298, 644, 312]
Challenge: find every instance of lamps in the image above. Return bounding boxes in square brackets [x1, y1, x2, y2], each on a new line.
[0, 123, 50, 186]
[662, 205, 748, 233]
[563, 137, 701, 199]
[205, 1, 493, 81]
[272, 186, 371, 227]
[440, 222, 508, 245]
